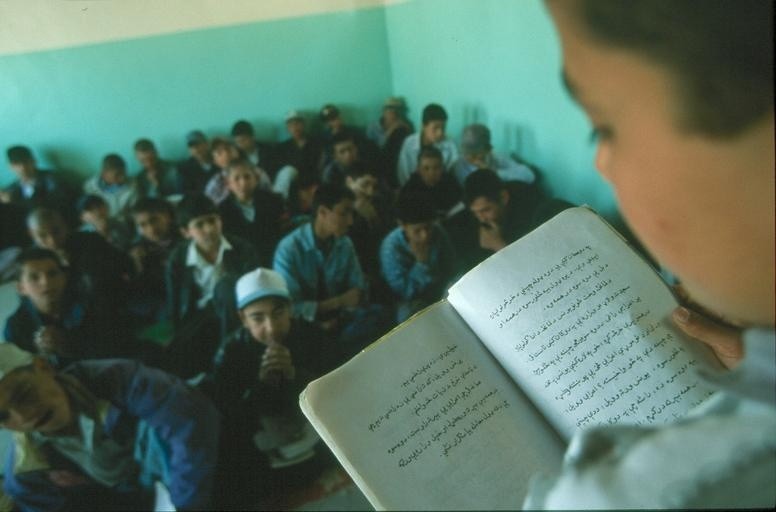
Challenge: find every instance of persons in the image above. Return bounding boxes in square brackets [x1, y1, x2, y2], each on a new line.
[522, 0, 775, 512]
[0, 97, 582, 512]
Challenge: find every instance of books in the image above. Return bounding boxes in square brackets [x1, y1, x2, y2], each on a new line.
[299, 204, 734, 512]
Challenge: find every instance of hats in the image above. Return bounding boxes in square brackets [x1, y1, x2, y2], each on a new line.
[1, 341, 36, 384]
[287, 110, 304, 125]
[320, 106, 339, 122]
[186, 130, 206, 146]
[234, 267, 291, 310]
